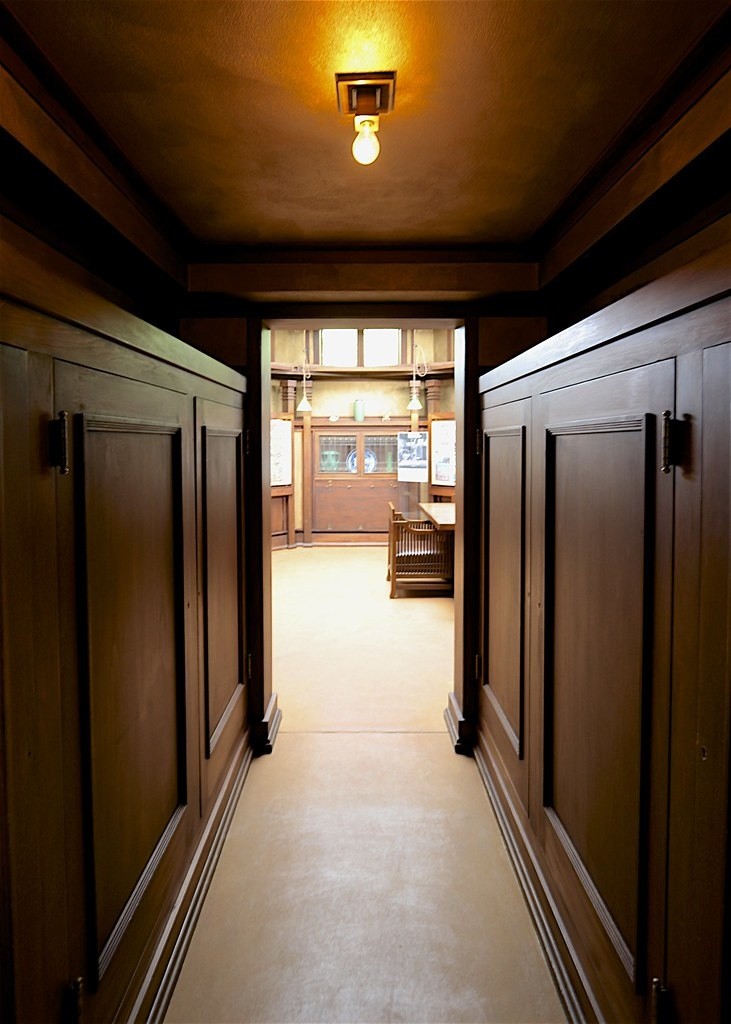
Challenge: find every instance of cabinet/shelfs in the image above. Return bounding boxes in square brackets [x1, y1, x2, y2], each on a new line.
[461, 240, 731, 1023]
[0, 239, 257, 1024]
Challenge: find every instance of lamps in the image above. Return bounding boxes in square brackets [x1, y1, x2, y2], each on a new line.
[298, 369, 313, 412]
[408, 371, 423, 410]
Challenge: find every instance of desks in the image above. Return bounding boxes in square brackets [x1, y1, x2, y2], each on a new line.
[418, 503, 457, 531]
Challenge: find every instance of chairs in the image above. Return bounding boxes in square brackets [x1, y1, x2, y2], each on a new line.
[388, 502, 456, 599]
